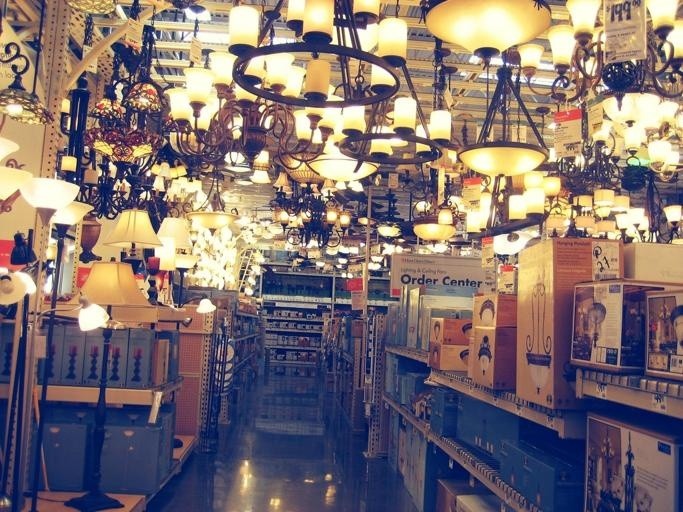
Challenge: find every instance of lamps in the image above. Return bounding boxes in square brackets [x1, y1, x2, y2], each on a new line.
[460, 350, 471, 366]
[0, 136, 30, 222]
[476, 336, 494, 377]
[594, 90, 683, 191]
[101, 208, 163, 279]
[153, 218, 195, 277]
[189, 219, 281, 298]
[422, 1, 547, 68]
[525, 275, 552, 397]
[67, 1, 181, 223]
[69, 261, 158, 503]
[333, 0, 452, 164]
[554, 139, 623, 199]
[433, 346, 438, 364]
[155, 235, 178, 286]
[33, 200, 93, 506]
[230, 3, 409, 108]
[585, 302, 607, 349]
[0, 176, 109, 506]
[516, 0, 683, 109]
[413, 178, 682, 259]
[461, 321, 472, 339]
[265, 151, 394, 280]
[452, 68, 550, 178]
[479, 299, 496, 325]
[150, 157, 212, 219]
[432, 321, 441, 339]
[163, 3, 338, 168]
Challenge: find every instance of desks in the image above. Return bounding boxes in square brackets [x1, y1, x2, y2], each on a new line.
[73, 433, 201, 511]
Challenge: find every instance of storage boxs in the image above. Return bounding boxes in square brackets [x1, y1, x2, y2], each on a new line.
[389, 253, 484, 299]
[472, 295, 517, 328]
[387, 303, 397, 345]
[436, 478, 483, 510]
[456, 494, 499, 512]
[395, 376, 402, 405]
[430, 386, 458, 437]
[455, 391, 558, 462]
[404, 421, 466, 512]
[569, 282, 665, 374]
[467, 326, 516, 391]
[421, 307, 458, 353]
[499, 438, 582, 510]
[406, 372, 428, 408]
[428, 343, 469, 371]
[384, 352, 423, 393]
[499, 265, 518, 295]
[407, 285, 478, 350]
[430, 317, 472, 344]
[516, 238, 625, 410]
[644, 289, 682, 381]
[583, 409, 680, 512]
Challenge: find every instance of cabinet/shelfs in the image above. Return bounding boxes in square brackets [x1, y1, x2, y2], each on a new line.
[264, 292, 335, 377]
[198, 287, 265, 451]
